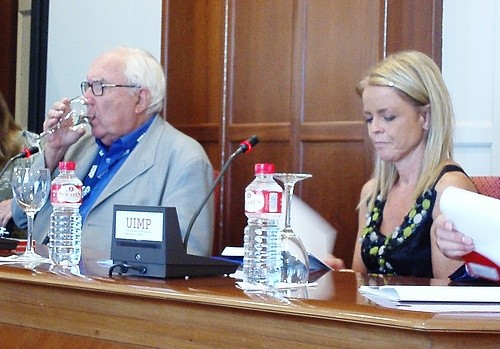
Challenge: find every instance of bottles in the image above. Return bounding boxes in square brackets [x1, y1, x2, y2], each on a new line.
[49, 161, 83, 268]
[242, 163, 283, 291]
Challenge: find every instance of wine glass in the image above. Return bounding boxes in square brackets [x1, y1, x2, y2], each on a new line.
[12, 167, 50, 263]
[22, 95, 97, 153]
[274, 173, 313, 288]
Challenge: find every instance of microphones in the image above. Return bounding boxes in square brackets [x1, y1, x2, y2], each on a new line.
[0, 147, 38, 179]
[111, 136, 258, 279]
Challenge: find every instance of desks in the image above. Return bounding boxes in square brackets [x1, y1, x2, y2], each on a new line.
[0, 251, 500, 349]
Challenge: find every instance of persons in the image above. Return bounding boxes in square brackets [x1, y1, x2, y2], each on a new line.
[323, 51, 500, 283]
[0, 48, 216, 256]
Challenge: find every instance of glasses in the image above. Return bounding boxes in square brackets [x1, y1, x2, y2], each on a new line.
[81, 81, 141, 96]
